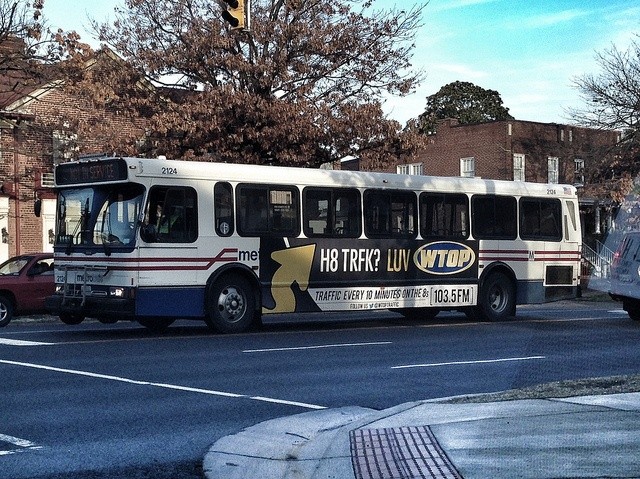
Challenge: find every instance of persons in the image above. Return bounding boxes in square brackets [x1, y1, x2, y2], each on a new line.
[37, 261, 49, 273]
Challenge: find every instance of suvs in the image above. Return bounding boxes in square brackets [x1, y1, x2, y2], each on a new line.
[607, 230, 640, 320]
[0, 253, 86, 326]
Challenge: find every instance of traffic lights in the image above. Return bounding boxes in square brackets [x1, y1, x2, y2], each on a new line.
[221, 0, 244, 30]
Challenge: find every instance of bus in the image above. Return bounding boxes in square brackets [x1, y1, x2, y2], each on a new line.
[33, 155, 584, 333]
